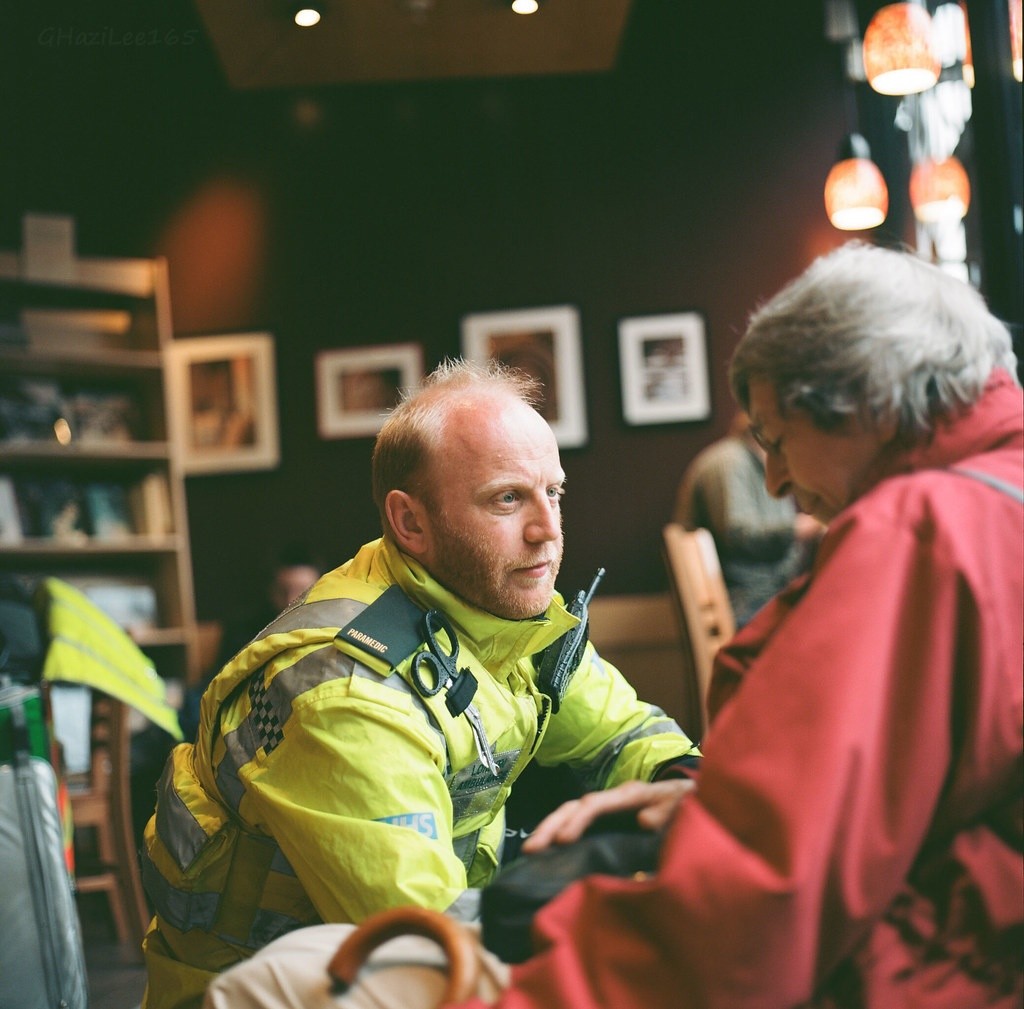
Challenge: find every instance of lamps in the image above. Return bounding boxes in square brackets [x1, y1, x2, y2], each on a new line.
[863, 0, 941, 96]
[894, 0, 974, 261]
[825, 82, 888, 230]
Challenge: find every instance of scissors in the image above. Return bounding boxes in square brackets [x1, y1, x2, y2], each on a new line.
[413, 608, 501, 779]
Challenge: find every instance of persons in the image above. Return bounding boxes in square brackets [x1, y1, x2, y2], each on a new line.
[677, 407, 832, 637]
[172, 556, 326, 725]
[145, 367, 705, 997]
[468, 243, 1024, 1008]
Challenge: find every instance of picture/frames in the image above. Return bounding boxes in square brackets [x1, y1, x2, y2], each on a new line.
[172, 331, 279, 476]
[316, 344, 424, 441]
[461, 304, 588, 451]
[619, 312, 709, 426]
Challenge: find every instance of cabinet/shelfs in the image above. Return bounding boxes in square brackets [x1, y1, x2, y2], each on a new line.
[0, 254, 198, 689]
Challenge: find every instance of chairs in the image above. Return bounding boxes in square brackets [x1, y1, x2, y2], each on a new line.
[662, 524, 734, 743]
[69, 687, 150, 960]
